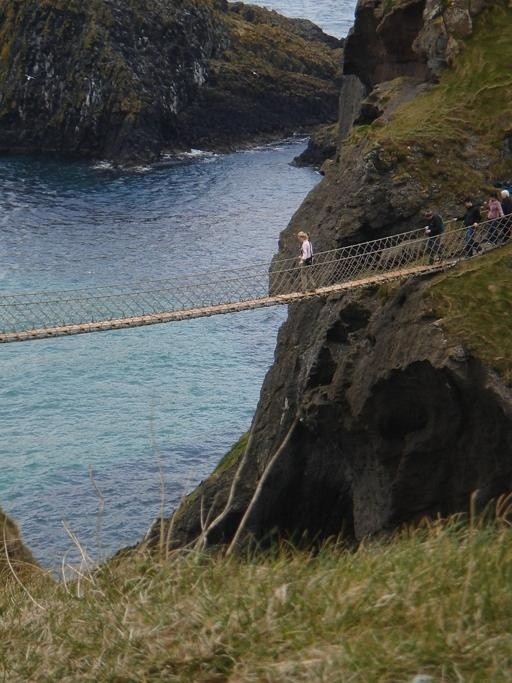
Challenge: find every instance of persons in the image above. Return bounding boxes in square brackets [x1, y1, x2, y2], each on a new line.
[296, 230, 317, 294]
[454, 196, 483, 258]
[498, 188, 512, 243]
[482, 192, 505, 246]
[422, 208, 445, 268]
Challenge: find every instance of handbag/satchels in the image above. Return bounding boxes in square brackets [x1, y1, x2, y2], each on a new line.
[304, 258, 312, 265]
